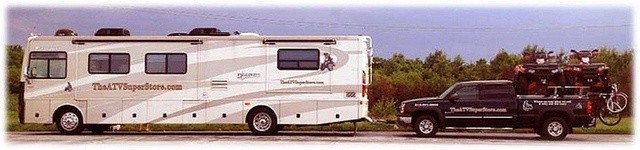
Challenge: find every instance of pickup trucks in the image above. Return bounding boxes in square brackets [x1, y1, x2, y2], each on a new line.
[396, 78, 594, 141]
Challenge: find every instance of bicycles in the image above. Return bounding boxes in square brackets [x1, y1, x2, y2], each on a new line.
[598, 77, 629, 126]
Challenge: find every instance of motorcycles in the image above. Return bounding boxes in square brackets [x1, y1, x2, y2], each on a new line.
[515, 50, 569, 99]
[564, 49, 610, 100]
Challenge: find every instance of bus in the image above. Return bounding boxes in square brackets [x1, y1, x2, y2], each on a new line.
[20, 26, 374, 136]
